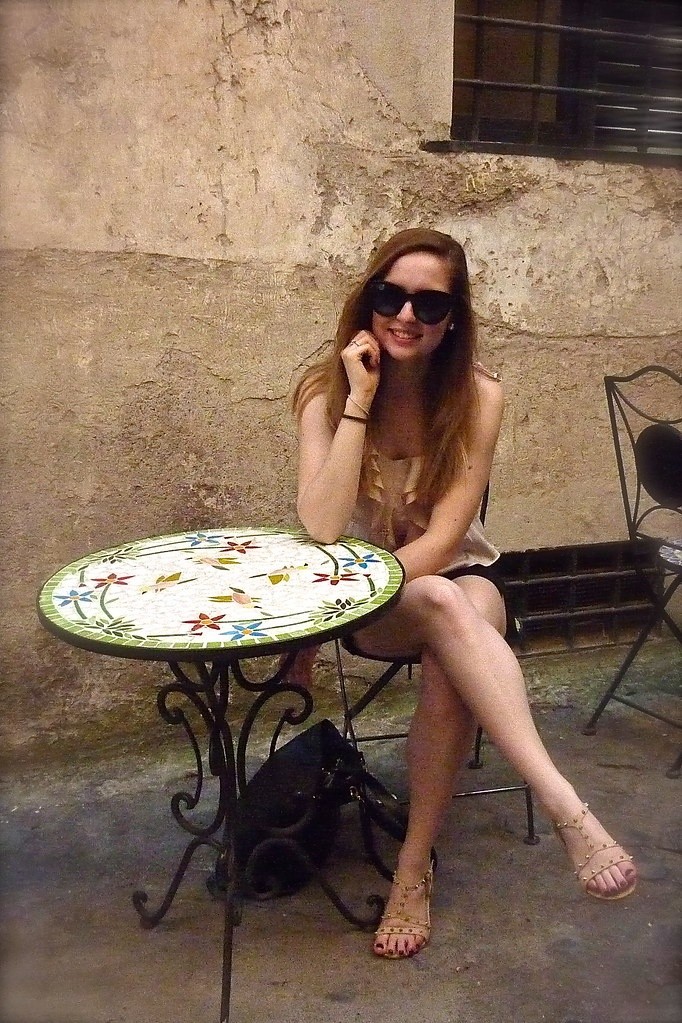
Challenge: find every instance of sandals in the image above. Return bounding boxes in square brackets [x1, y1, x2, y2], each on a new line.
[551, 801, 636, 900]
[373, 850, 434, 959]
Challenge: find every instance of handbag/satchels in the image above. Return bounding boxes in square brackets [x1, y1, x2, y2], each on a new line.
[216, 719, 438, 899]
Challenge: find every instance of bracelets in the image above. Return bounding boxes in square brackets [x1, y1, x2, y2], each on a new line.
[341, 413, 370, 423]
[347, 394, 370, 415]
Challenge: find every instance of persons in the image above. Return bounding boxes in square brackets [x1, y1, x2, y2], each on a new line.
[296, 229, 638, 958]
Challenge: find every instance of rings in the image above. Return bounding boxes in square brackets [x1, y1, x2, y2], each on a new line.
[351, 340, 360, 346]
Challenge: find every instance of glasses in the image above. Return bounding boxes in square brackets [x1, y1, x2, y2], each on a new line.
[369, 281, 455, 326]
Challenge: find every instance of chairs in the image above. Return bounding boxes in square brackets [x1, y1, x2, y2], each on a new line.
[587, 366, 682, 779]
[336, 478, 541, 876]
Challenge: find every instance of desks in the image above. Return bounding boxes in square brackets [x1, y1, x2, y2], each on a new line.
[35, 526, 438, 1023]
